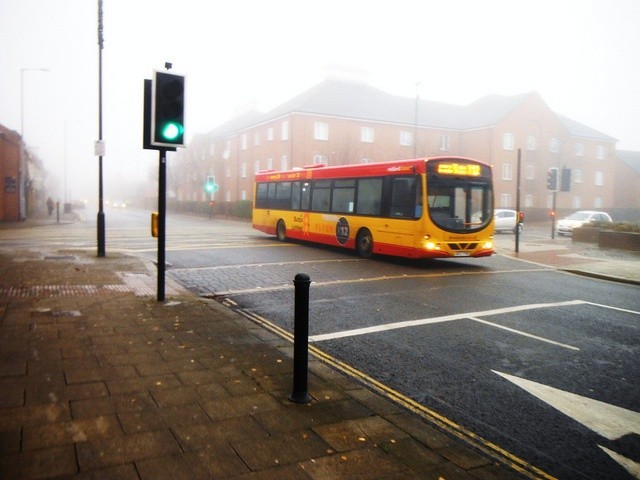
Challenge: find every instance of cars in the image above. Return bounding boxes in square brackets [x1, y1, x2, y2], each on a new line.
[555, 211, 613, 237]
[470, 208, 524, 234]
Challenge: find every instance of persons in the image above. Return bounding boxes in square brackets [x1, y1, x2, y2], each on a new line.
[47, 196, 54, 216]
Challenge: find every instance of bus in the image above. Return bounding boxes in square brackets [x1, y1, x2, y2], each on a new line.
[252, 157, 496, 259]
